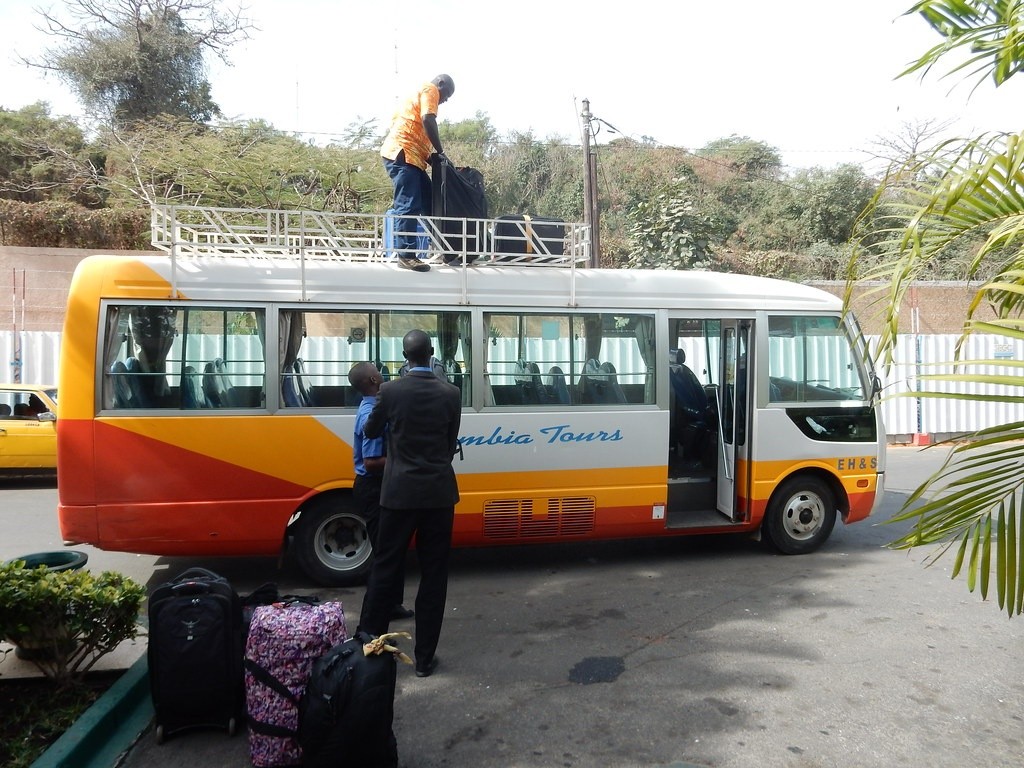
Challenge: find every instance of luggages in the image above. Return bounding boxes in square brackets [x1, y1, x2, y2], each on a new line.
[491, 211, 565, 265]
[382, 209, 429, 258]
[147, 566, 243, 746]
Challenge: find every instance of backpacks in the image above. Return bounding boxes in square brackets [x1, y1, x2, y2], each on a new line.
[298, 629, 399, 768]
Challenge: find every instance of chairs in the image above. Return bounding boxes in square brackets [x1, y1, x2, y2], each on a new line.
[109, 350, 632, 407]
[668, 346, 720, 438]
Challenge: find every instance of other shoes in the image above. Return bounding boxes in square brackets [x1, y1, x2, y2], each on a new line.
[398, 256, 430, 272]
[417, 655, 438, 677]
[390, 605, 414, 619]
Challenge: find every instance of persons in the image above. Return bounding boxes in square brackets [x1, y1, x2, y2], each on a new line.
[379, 74, 457, 272]
[25, 395, 46, 417]
[347, 361, 415, 620]
[353, 329, 464, 679]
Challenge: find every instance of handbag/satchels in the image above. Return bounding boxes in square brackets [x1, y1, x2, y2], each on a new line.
[245, 593, 350, 768]
[234, 578, 322, 731]
[430, 152, 487, 266]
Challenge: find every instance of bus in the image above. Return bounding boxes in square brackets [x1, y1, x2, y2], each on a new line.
[57, 199, 891, 591]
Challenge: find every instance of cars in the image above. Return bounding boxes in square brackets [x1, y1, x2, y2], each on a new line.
[0, 383, 59, 476]
